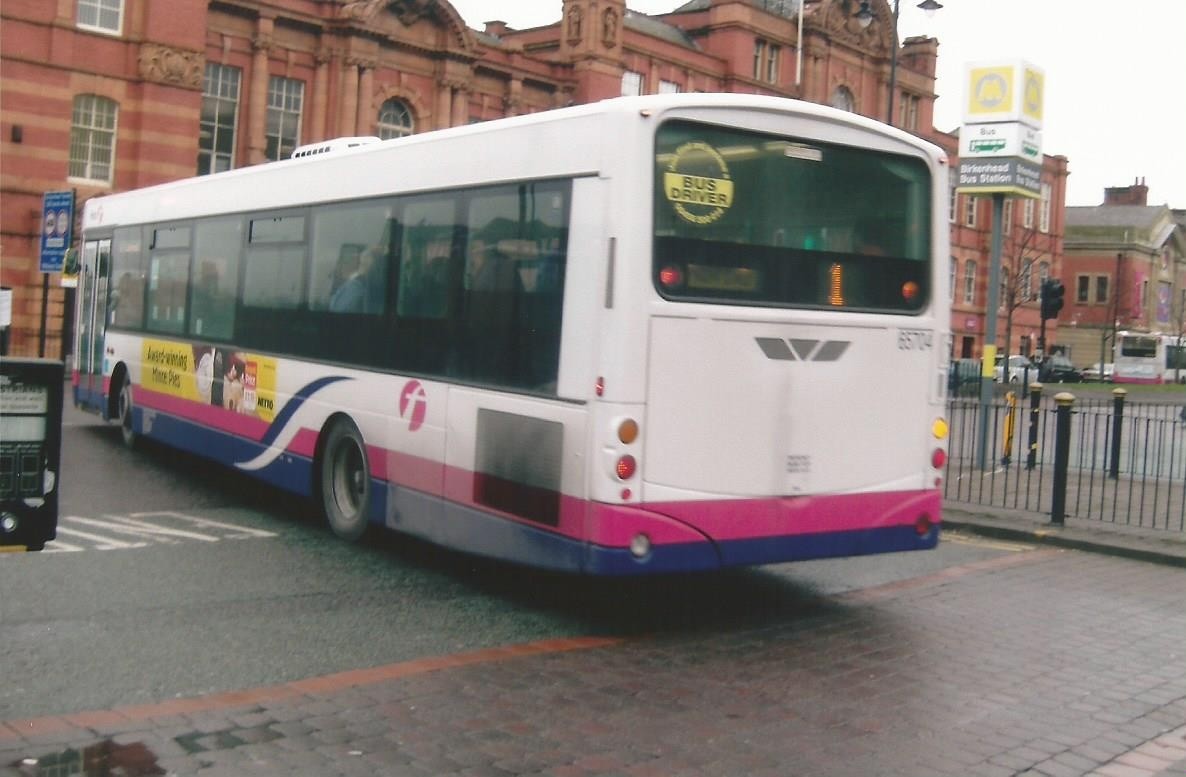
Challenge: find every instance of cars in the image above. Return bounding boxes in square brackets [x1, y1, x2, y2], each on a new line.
[1077, 362, 1114, 382]
[949, 357, 981, 391]
[992, 354, 1041, 387]
[1025, 354, 1085, 385]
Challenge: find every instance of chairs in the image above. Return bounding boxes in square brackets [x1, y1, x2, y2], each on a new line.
[408, 249, 580, 315]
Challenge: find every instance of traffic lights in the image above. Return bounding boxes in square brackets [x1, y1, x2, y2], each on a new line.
[1041, 277, 1066, 321]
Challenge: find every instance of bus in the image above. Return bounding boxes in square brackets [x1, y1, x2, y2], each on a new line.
[56, 89, 954, 583]
[1111, 328, 1186, 386]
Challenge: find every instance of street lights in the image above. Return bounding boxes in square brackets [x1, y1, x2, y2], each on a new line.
[886, 0, 945, 126]
